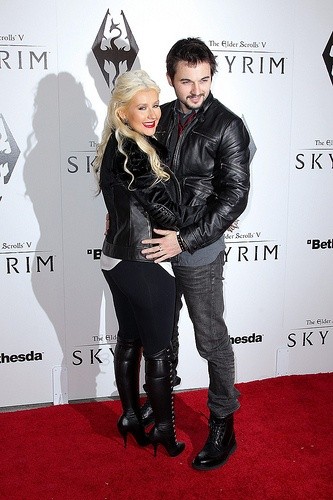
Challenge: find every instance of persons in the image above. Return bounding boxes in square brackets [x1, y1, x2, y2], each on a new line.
[102, 38, 250, 471]
[92, 70, 220, 459]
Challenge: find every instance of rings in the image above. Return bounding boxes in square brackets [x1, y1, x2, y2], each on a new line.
[159, 245, 161, 249]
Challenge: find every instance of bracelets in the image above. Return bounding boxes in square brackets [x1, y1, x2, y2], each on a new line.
[176, 231, 185, 252]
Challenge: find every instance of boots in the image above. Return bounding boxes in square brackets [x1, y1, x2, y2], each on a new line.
[140, 399, 155, 430]
[191, 414, 237, 470]
[113, 341, 185, 458]
